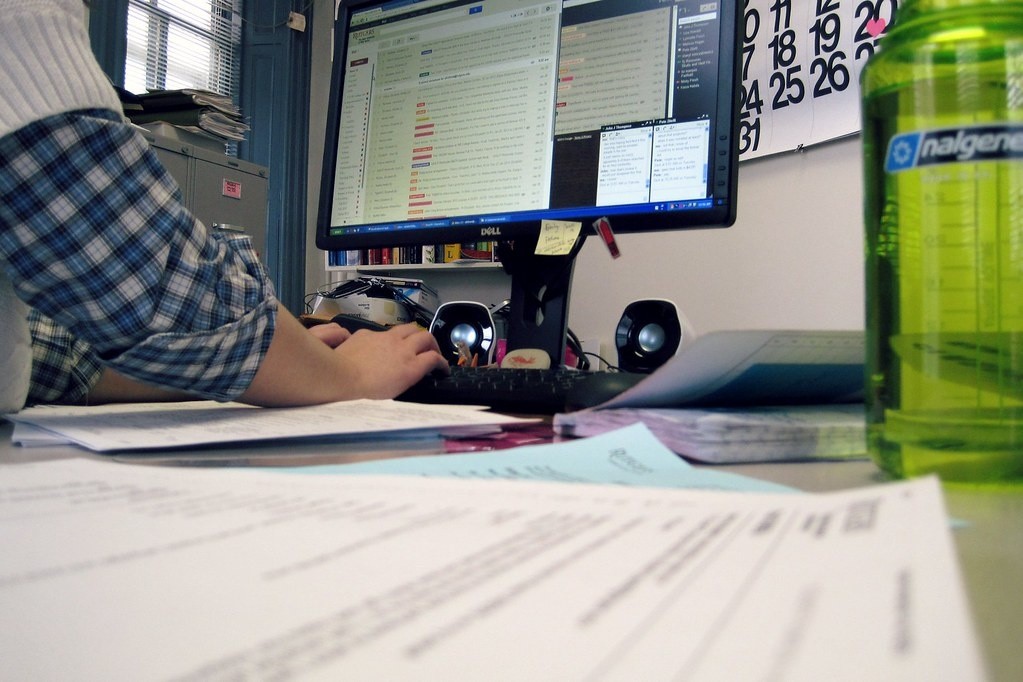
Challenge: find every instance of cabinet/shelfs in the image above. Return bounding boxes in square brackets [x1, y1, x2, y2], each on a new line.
[131, 128, 269, 268]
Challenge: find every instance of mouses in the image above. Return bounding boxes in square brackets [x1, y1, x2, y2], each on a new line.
[562, 373, 649, 413]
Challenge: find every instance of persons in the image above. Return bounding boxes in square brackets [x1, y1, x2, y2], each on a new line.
[0, 0, 449, 416]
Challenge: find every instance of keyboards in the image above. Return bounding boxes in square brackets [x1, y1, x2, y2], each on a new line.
[394, 365, 603, 415]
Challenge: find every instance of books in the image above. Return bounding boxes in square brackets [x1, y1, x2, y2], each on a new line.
[554, 328, 873, 461]
[122, 88, 251, 144]
[361, 275, 438, 296]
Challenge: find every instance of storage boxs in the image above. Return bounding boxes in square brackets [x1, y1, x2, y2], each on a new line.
[146, 125, 227, 152]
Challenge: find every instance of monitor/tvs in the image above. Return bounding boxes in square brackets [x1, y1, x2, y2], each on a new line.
[314, 0, 745, 369]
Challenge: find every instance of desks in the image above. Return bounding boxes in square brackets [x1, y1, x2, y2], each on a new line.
[0, 420, 1023, 682]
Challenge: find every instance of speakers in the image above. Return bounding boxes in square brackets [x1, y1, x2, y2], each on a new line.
[427, 301, 496, 369]
[614, 298, 698, 376]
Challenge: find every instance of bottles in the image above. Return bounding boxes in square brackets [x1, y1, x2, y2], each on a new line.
[859, 0, 1023, 484]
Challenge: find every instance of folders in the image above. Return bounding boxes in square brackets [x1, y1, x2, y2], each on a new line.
[129, 88, 245, 139]
